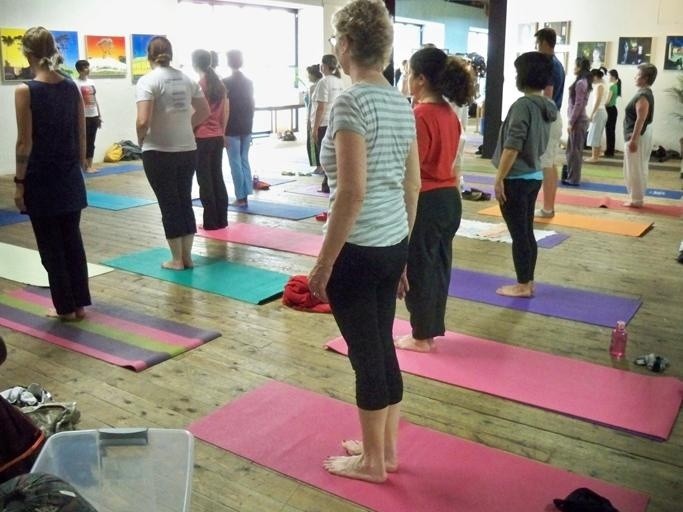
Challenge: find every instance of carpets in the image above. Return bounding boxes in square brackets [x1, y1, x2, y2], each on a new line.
[86, 191, 156, 211]
[188, 378, 650, 512]
[461, 165, 497, 175]
[0, 243, 115, 287]
[461, 184, 496, 201]
[196, 220, 324, 259]
[260, 175, 295, 186]
[284, 184, 333, 197]
[80, 165, 143, 181]
[1, 287, 221, 373]
[478, 203, 653, 237]
[537, 191, 683, 220]
[193, 196, 324, 219]
[325, 318, 683, 442]
[455, 219, 570, 250]
[557, 178, 682, 200]
[0, 210, 30, 226]
[448, 268, 643, 329]
[101, 246, 291, 303]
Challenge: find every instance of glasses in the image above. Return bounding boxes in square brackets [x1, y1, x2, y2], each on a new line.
[327, 35, 336, 46]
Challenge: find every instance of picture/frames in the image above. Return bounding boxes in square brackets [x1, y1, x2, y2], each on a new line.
[85, 35, 127, 79]
[543, 20, 570, 45]
[131, 34, 166, 84]
[575, 42, 608, 69]
[0, 28, 79, 82]
[662, 34, 682, 74]
[554, 51, 568, 76]
[616, 36, 655, 67]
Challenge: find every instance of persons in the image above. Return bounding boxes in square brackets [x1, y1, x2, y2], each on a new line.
[583, 67, 607, 163]
[221, 50, 254, 206]
[598, 69, 620, 157]
[621, 63, 657, 207]
[208, 50, 218, 68]
[73, 60, 101, 174]
[307, 0, 421, 484]
[560, 57, 593, 186]
[533, 27, 564, 217]
[392, 47, 476, 352]
[190, 48, 229, 230]
[135, 36, 210, 270]
[13, 25, 92, 321]
[304, 55, 468, 195]
[490, 51, 556, 297]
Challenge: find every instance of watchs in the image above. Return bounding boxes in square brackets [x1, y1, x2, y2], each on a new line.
[14, 176, 25, 184]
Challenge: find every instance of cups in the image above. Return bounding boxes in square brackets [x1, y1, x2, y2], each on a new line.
[609, 321, 628, 357]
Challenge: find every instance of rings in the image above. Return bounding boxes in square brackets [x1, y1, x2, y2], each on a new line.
[312, 290, 319, 298]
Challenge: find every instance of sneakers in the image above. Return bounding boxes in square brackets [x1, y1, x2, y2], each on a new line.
[462, 188, 491, 200]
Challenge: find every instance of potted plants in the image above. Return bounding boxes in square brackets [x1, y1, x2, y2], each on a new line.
[663, 75, 683, 159]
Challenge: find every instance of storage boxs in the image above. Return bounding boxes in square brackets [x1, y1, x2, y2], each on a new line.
[30, 427, 195, 512]
